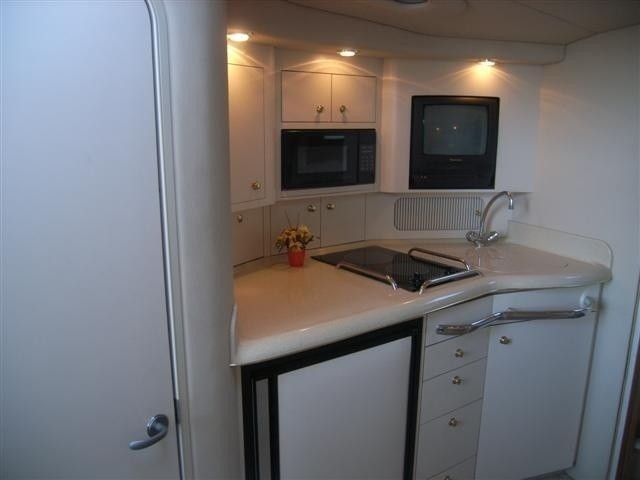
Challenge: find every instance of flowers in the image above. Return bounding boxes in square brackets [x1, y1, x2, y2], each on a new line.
[273, 209, 320, 255]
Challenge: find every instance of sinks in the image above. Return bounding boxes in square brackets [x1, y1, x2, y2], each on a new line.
[466, 244, 561, 274]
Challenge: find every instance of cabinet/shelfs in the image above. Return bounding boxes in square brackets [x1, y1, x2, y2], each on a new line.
[232, 208, 263, 267]
[227, 64, 266, 203]
[281, 71, 382, 122]
[270, 194, 365, 255]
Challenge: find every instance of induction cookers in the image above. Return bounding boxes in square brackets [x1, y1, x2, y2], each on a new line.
[309, 242, 477, 294]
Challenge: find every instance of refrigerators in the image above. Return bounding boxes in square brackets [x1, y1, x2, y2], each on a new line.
[0, 0, 247, 480]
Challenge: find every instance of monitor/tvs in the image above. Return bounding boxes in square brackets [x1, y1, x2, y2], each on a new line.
[409, 95, 500, 190]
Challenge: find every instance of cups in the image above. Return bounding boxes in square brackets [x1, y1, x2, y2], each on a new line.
[286, 246, 305, 267]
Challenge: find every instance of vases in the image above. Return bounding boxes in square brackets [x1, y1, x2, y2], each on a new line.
[288, 248, 305, 267]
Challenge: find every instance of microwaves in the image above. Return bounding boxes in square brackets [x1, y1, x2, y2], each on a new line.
[278, 122, 380, 198]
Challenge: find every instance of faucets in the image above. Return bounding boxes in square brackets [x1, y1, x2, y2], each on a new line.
[479, 190, 513, 239]
